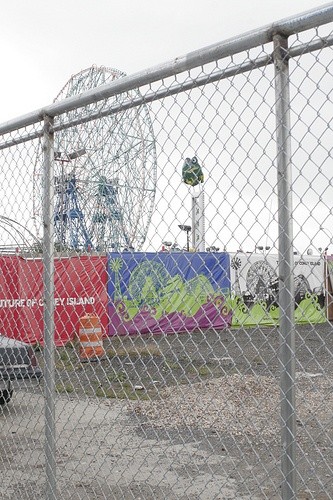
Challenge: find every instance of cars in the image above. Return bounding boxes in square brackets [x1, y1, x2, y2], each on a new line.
[0, 337, 42, 405]
